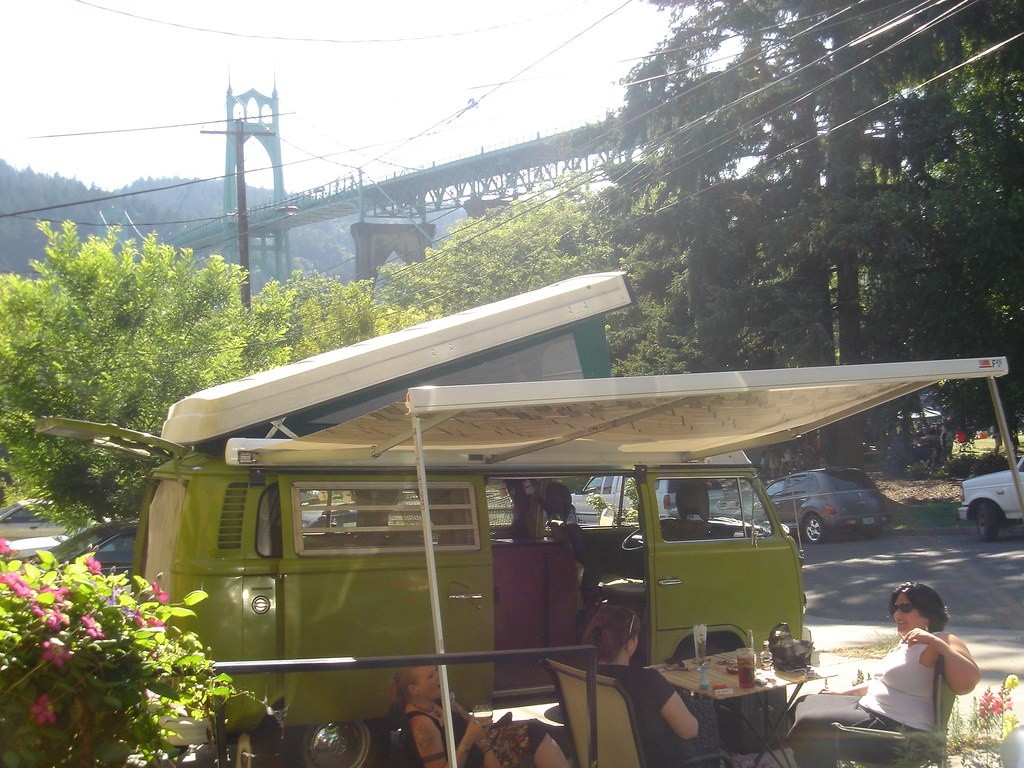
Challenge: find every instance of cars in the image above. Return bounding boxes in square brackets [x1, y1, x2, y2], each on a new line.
[26, 516, 139, 586]
[301, 500, 357, 530]
[958, 455, 1023, 542]
[752, 464, 894, 545]
[1, 496, 94, 540]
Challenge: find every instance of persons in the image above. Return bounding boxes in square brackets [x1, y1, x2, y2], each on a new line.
[786, 582, 982, 768]
[545, 482, 581, 560]
[391, 665, 569, 768]
[582, 604, 722, 768]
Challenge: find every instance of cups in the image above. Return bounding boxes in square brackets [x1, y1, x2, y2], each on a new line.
[736, 648, 754, 688]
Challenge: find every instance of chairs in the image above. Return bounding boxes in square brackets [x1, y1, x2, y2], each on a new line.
[544, 483, 647, 612]
[546, 660, 732, 768]
[831, 655, 956, 768]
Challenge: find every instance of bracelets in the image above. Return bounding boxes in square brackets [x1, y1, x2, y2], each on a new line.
[458, 745, 471, 752]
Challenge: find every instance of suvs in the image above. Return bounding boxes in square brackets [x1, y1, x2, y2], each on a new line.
[570, 449, 764, 528]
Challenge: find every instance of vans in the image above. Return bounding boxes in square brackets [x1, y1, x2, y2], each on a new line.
[35, 267, 1024, 768]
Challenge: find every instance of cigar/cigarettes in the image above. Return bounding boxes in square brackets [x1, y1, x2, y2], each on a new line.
[898, 633, 908, 644]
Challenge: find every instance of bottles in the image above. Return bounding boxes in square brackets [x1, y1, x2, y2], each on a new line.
[760, 640, 774, 680]
[746, 629, 756, 675]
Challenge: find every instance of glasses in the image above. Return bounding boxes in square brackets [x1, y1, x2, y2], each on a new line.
[891, 601, 914, 613]
[621, 606, 637, 635]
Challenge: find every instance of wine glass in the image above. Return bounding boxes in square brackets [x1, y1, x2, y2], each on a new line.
[473, 704, 493, 747]
[693, 625, 707, 671]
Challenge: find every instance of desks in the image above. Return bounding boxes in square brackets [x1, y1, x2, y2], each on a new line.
[642, 645, 839, 768]
